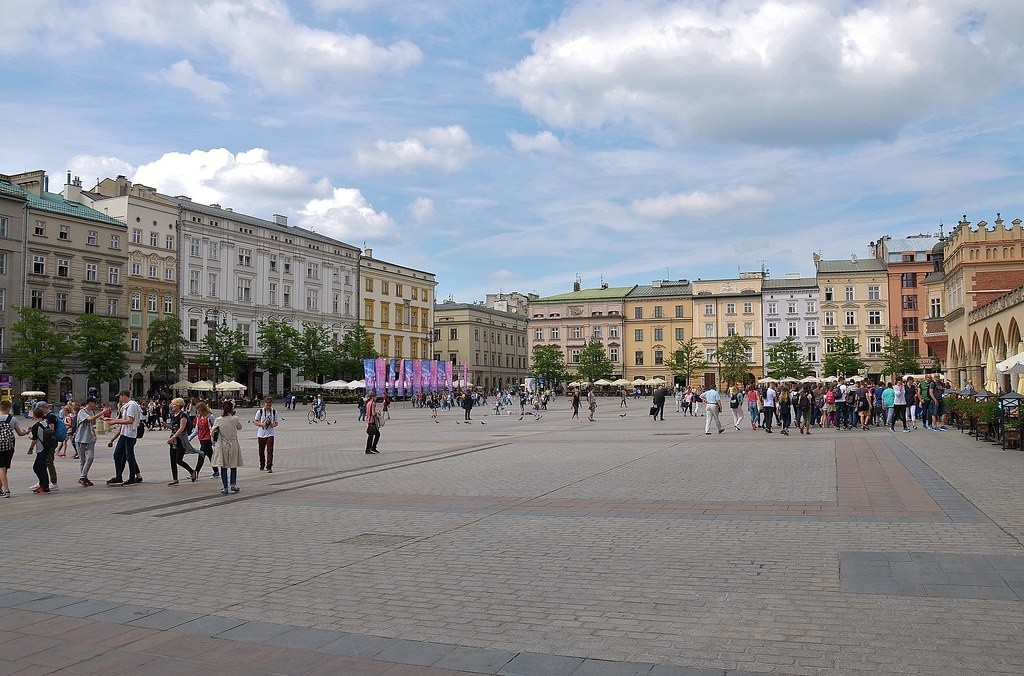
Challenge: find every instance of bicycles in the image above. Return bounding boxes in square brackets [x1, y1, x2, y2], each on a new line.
[307, 404, 326, 422]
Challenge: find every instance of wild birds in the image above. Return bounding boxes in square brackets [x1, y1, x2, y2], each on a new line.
[281, 417, 285, 421]
[535, 417, 540, 421]
[334, 420, 336, 424]
[435, 421, 439, 423]
[481, 421, 486, 425]
[518, 416, 523, 420]
[308, 420, 317, 424]
[464, 421, 471, 425]
[619, 412, 627, 418]
[247, 420, 250, 423]
[326, 421, 330, 425]
[456, 421, 460, 424]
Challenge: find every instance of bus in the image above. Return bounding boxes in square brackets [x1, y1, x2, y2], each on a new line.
[0, 387, 13, 403]
[520, 384, 525, 395]
[475, 386, 483, 397]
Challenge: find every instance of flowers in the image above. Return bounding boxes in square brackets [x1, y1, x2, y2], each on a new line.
[942, 395, 1023, 428]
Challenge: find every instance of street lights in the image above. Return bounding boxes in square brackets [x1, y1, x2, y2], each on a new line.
[591, 339, 598, 382]
[210, 353, 219, 400]
[313, 329, 330, 384]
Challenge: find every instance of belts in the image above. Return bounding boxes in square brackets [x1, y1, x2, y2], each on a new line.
[707, 403, 718, 404]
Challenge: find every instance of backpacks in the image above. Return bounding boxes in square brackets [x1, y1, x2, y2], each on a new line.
[0, 414, 16, 451]
[36, 422, 58, 449]
[826, 389, 835, 404]
[799, 394, 811, 412]
[47, 413, 67, 442]
[730, 392, 740, 409]
[172, 412, 193, 436]
[833, 385, 842, 399]
[847, 380, 945, 412]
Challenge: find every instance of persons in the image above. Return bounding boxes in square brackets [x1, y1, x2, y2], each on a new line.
[253, 396, 279, 473]
[0, 388, 279, 498]
[917, 374, 933, 430]
[284, 392, 390, 422]
[364, 392, 381, 454]
[929, 373, 950, 431]
[209, 401, 244, 494]
[410, 386, 666, 422]
[675, 376, 974, 436]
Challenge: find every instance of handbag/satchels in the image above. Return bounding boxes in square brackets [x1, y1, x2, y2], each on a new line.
[650, 403, 658, 416]
[367, 424, 377, 435]
[136, 423, 144, 438]
[213, 426, 219, 442]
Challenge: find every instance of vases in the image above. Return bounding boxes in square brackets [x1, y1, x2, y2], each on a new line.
[1004, 428, 1020, 440]
[975, 422, 987, 432]
[961, 418, 969, 426]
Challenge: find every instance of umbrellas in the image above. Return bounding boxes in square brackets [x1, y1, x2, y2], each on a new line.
[758, 374, 869, 388]
[294, 379, 474, 396]
[569, 378, 668, 396]
[996, 351, 1024, 374]
[169, 380, 247, 410]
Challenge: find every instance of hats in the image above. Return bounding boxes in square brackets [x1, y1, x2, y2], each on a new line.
[36, 401, 48, 408]
[136, 395, 167, 408]
[708, 384, 717, 389]
[87, 396, 98, 403]
[115, 389, 130, 397]
[803, 387, 809, 393]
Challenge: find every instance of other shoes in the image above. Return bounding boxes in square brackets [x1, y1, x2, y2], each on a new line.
[0, 448, 277, 502]
[144, 424, 171, 432]
[387, 402, 956, 434]
